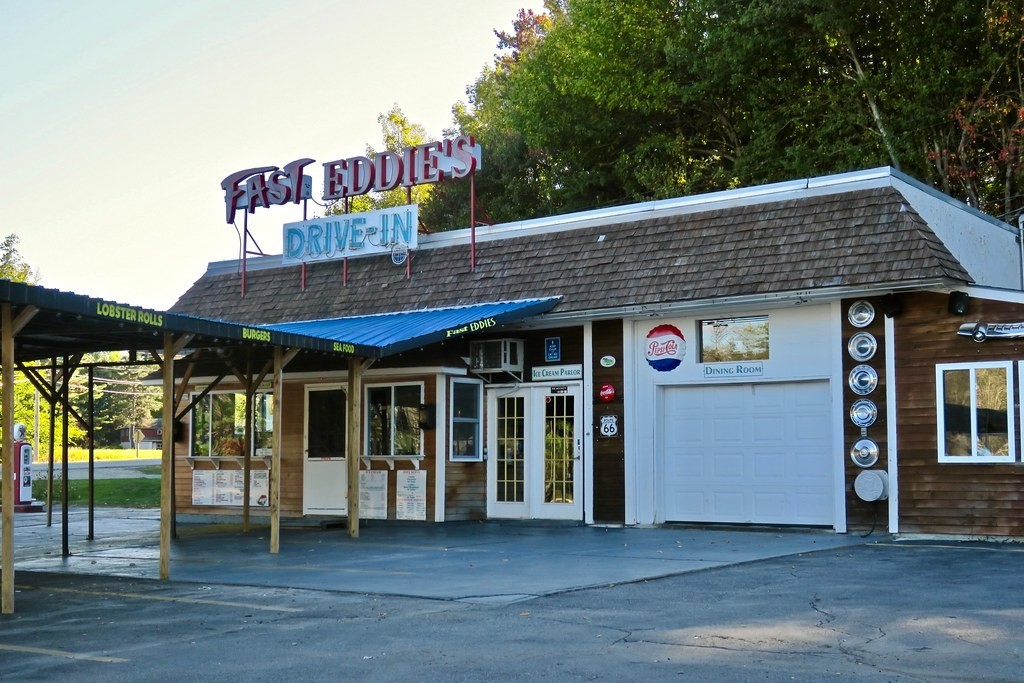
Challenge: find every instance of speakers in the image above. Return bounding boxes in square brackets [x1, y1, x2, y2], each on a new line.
[876, 294, 903, 318]
[948, 291, 968, 315]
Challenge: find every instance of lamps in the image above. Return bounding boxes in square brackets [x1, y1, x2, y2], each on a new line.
[947, 291, 969, 316]
[419, 404, 430, 430]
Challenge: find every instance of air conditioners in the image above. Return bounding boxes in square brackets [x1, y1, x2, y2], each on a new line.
[469, 338, 523, 373]
